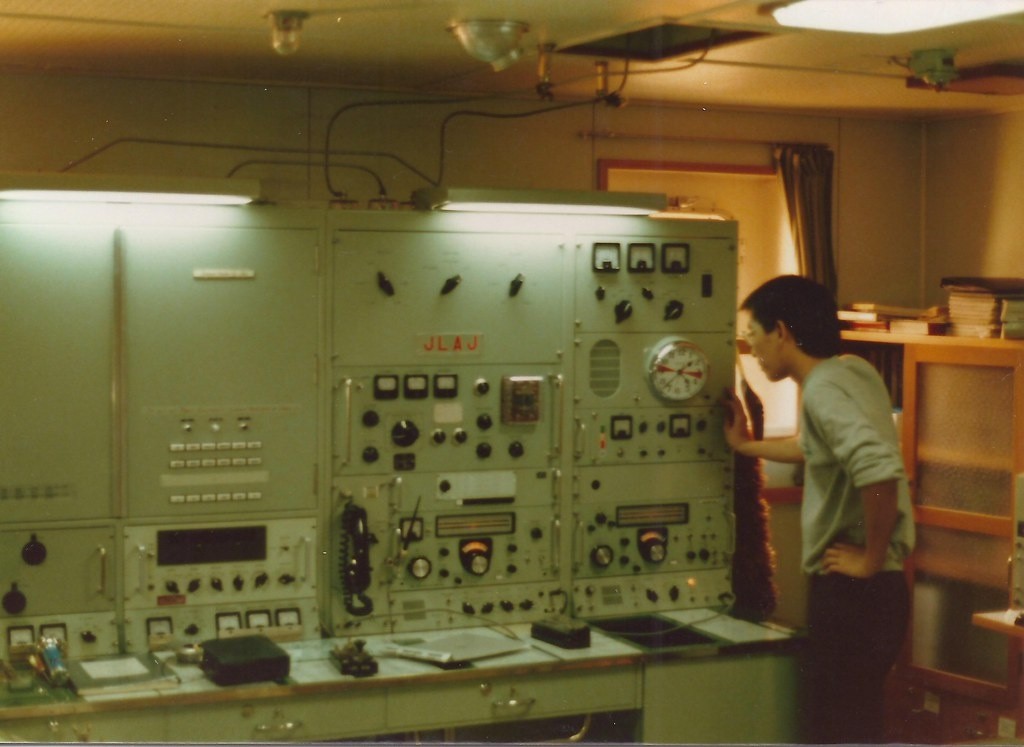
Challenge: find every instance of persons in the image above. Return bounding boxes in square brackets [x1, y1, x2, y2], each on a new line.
[718, 274, 917, 743]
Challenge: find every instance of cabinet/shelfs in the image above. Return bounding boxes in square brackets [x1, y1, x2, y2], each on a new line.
[837, 330, 1024, 747]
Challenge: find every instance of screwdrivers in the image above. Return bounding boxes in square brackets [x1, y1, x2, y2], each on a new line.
[28, 653, 56, 687]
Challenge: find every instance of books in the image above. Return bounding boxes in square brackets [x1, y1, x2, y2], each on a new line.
[836, 276, 1024, 339]
[67, 652, 182, 696]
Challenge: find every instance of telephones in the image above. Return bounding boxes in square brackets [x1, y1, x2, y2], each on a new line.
[341, 505, 373, 595]
